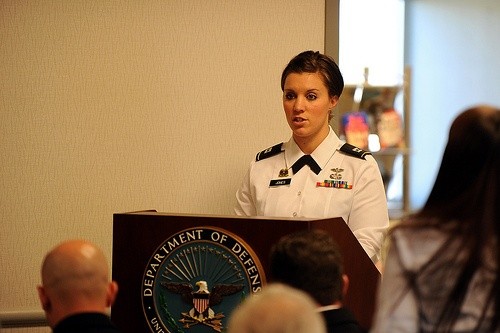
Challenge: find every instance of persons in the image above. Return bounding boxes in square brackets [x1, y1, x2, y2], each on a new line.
[232, 49, 390, 265]
[370, 101, 500, 333]
[37, 238, 125, 333]
[262, 228, 369, 333]
[224, 283, 326, 333]
